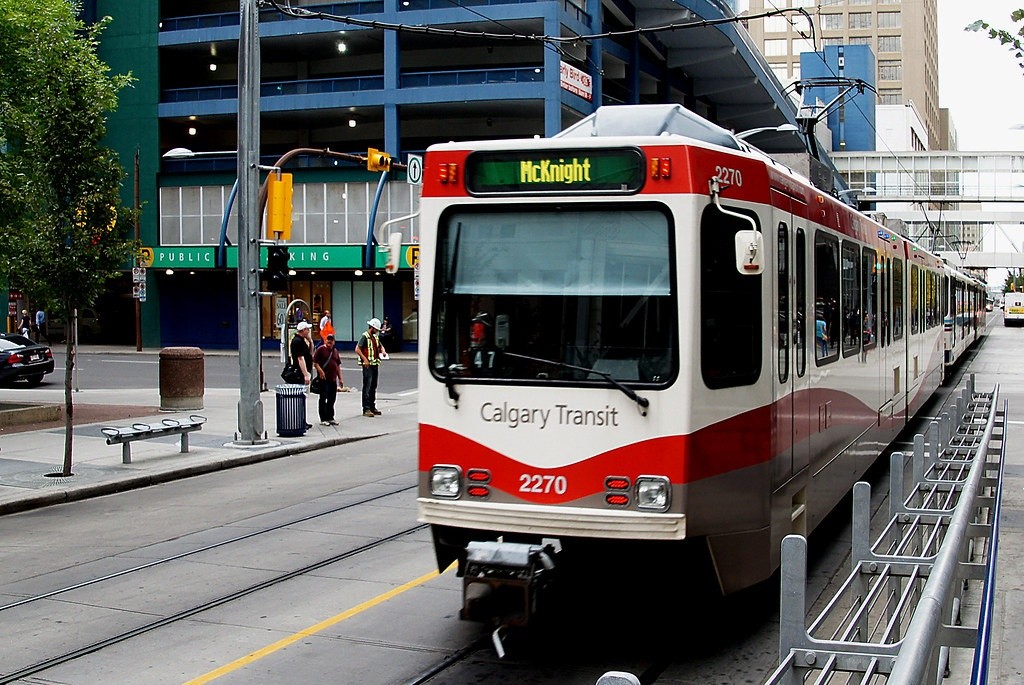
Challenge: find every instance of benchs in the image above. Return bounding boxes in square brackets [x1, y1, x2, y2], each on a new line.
[101, 415, 207, 465]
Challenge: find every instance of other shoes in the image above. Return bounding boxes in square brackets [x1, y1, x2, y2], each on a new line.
[306, 423, 312, 428]
[306, 426, 309, 429]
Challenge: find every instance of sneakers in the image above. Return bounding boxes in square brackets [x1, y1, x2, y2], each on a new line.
[329, 420, 339, 425]
[363, 410, 374, 416]
[320, 421, 330, 425]
[371, 409, 381, 415]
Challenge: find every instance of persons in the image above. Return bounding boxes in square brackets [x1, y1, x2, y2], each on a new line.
[291, 322, 315, 430]
[35, 305, 52, 346]
[842, 308, 860, 346]
[312, 334, 344, 426]
[380, 317, 392, 350]
[863, 313, 871, 346]
[355, 318, 386, 417]
[816, 314, 828, 357]
[320, 310, 336, 344]
[18, 310, 31, 339]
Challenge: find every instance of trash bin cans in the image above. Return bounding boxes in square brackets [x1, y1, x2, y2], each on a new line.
[276, 384, 308, 437]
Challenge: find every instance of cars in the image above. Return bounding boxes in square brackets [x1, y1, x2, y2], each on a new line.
[0, 333, 54, 387]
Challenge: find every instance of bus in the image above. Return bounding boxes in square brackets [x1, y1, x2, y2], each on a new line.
[415, 102, 988, 659]
[985, 298, 993, 312]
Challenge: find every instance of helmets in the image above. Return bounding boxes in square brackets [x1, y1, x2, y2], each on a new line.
[367, 318, 381, 330]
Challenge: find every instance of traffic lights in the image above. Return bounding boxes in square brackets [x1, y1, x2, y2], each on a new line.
[367, 147, 392, 172]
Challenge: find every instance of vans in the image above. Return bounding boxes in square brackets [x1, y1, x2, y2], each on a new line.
[1000, 292, 1024, 326]
[999, 298, 1005, 309]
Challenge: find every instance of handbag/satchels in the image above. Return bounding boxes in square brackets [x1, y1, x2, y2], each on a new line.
[280, 364, 305, 385]
[310, 377, 323, 394]
[822, 333, 828, 341]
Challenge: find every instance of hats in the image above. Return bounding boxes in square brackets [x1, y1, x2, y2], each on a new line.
[22, 310, 27, 314]
[296, 321, 312, 330]
[327, 334, 335, 339]
